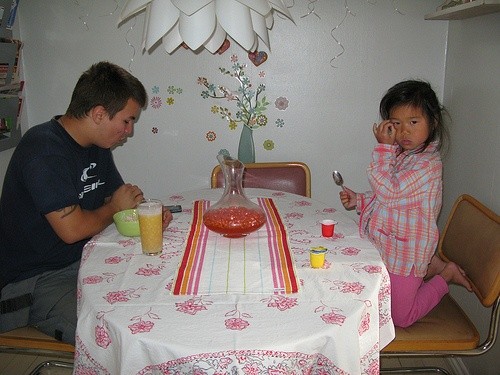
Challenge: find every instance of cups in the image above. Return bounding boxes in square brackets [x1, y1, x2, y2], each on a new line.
[137, 199, 163, 256]
[307, 246, 328, 268]
[319, 219, 338, 237]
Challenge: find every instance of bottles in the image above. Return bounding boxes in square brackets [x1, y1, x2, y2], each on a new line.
[203, 154, 266, 239]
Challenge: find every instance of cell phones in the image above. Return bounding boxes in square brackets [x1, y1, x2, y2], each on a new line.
[165, 205, 182, 213]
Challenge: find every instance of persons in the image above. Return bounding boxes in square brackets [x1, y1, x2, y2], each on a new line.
[0, 60, 174, 346]
[338, 79, 475, 328]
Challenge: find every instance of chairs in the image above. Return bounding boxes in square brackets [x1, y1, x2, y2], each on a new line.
[211, 162, 311, 198]
[380, 195, 500, 375]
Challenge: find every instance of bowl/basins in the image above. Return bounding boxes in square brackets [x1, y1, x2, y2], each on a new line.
[113, 209, 140, 236]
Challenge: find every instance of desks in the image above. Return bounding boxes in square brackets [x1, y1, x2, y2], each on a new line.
[74, 189, 396, 375]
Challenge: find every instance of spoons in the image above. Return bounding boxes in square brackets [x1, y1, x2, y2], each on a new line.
[333, 171, 351, 199]
